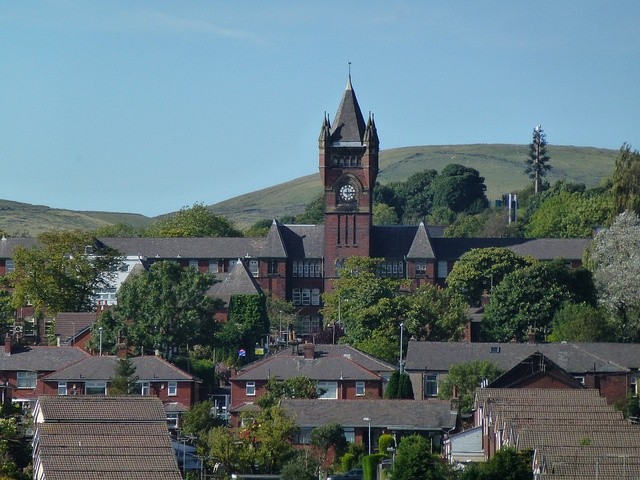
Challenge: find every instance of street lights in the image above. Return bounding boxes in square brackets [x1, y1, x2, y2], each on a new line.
[98, 326, 103, 355]
[399, 321, 403, 374]
[362, 416, 372, 455]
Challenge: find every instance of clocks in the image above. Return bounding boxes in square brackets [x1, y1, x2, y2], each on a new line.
[339, 183, 356, 203]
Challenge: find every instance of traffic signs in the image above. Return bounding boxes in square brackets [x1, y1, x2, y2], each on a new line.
[254, 347, 264, 354]
[238, 349, 246, 356]
